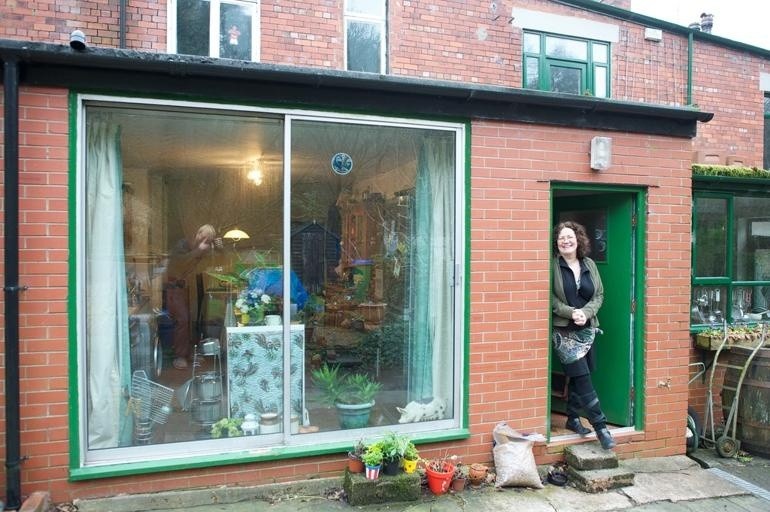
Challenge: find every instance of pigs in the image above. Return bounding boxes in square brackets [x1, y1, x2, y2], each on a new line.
[396, 395, 448, 424]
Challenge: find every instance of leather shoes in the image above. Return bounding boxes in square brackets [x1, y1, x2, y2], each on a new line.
[564, 416, 591, 435]
[594, 426, 618, 450]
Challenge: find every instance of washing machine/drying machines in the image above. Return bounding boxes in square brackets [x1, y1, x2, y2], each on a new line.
[129, 313, 163, 378]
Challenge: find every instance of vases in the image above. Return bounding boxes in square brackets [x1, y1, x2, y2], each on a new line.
[249, 303, 264, 323]
[453, 478, 465, 491]
[427, 463, 454, 494]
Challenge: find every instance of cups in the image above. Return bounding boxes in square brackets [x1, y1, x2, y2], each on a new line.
[265, 313, 281, 327]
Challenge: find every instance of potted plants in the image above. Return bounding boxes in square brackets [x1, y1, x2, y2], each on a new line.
[312, 360, 383, 430]
[346, 434, 366, 473]
[402, 442, 419, 473]
[381, 431, 404, 475]
[360, 448, 383, 481]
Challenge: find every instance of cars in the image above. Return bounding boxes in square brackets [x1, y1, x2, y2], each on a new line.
[744, 307, 769, 321]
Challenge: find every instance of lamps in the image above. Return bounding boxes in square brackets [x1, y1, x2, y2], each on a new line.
[219, 222, 251, 242]
[590, 135, 613, 171]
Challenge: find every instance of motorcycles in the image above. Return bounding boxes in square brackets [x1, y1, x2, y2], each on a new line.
[691, 292, 724, 323]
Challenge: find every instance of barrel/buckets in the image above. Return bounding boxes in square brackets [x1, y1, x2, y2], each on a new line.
[159, 317, 177, 344]
[722, 345, 770, 459]
[304, 303, 326, 328]
[352, 258, 375, 284]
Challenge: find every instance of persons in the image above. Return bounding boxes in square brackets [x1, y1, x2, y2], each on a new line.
[552, 221, 616, 450]
[164, 224, 216, 370]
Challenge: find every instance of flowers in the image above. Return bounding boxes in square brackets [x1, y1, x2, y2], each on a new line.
[234, 287, 272, 324]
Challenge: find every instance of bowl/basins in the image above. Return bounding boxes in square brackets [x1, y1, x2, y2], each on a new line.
[742, 312, 763, 322]
[693, 304, 706, 323]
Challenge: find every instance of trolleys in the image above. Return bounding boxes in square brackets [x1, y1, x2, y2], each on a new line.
[684, 311, 767, 457]
